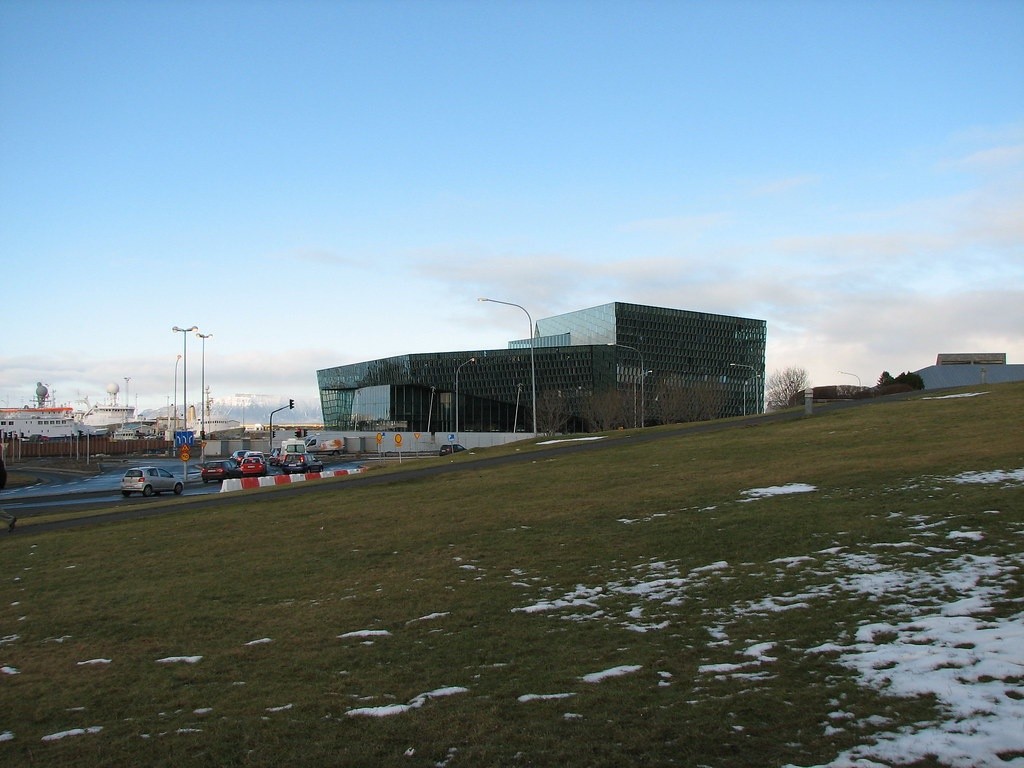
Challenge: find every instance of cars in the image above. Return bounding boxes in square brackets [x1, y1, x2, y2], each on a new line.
[239, 456, 267, 476]
[268, 447, 281, 466]
[229, 450, 250, 464]
[281, 452, 323, 475]
[200, 459, 243, 483]
[439, 444, 467, 455]
[244, 451, 266, 462]
[3, 430, 49, 443]
[120, 465, 184, 497]
[91, 426, 166, 440]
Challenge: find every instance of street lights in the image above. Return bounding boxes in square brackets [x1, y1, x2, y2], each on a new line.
[195, 332, 213, 439]
[190, 405, 196, 421]
[743, 374, 762, 416]
[454, 357, 474, 432]
[733, 363, 759, 416]
[513, 381, 524, 434]
[123, 377, 131, 406]
[173, 325, 198, 431]
[632, 370, 655, 428]
[476, 296, 538, 438]
[836, 370, 862, 386]
[167, 403, 174, 430]
[606, 340, 646, 430]
[173, 353, 183, 437]
[426, 385, 436, 433]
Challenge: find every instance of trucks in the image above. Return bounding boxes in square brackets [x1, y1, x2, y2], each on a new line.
[244, 423, 264, 435]
[304, 439, 346, 456]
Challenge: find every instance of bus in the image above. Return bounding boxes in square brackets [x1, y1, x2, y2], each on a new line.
[113, 432, 139, 440]
[279, 437, 307, 464]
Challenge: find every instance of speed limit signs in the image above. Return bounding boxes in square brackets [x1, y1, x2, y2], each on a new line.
[181, 453, 189, 461]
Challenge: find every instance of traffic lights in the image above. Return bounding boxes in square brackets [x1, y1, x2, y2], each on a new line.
[304, 429, 308, 437]
[290, 399, 294, 409]
[294, 429, 301, 437]
[272, 431, 276, 438]
